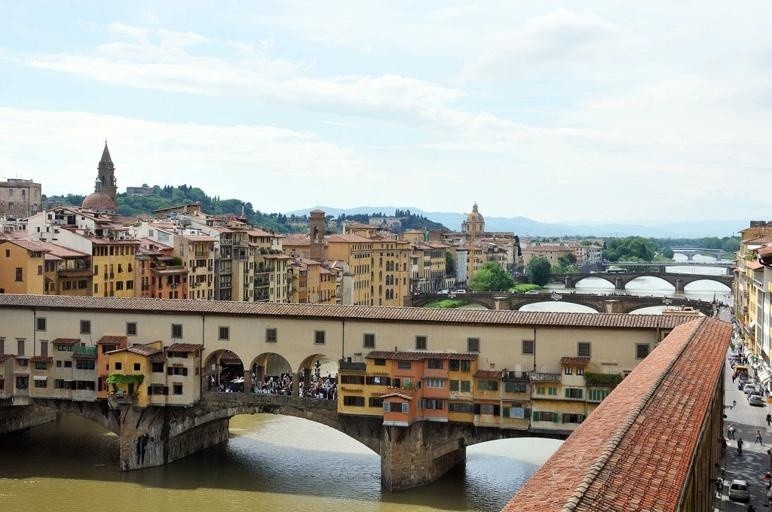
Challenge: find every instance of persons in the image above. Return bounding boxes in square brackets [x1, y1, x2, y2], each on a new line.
[209, 369, 338, 401]
[715, 353, 772, 512]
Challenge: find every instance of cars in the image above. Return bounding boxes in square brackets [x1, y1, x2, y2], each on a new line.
[525, 290, 539, 294]
[728, 354, 762, 406]
[728, 479, 749, 501]
[437, 289, 466, 294]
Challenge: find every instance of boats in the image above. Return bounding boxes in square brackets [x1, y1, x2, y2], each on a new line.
[662, 307, 699, 315]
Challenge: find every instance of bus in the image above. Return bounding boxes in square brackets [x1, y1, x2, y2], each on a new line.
[607, 269, 627, 274]
